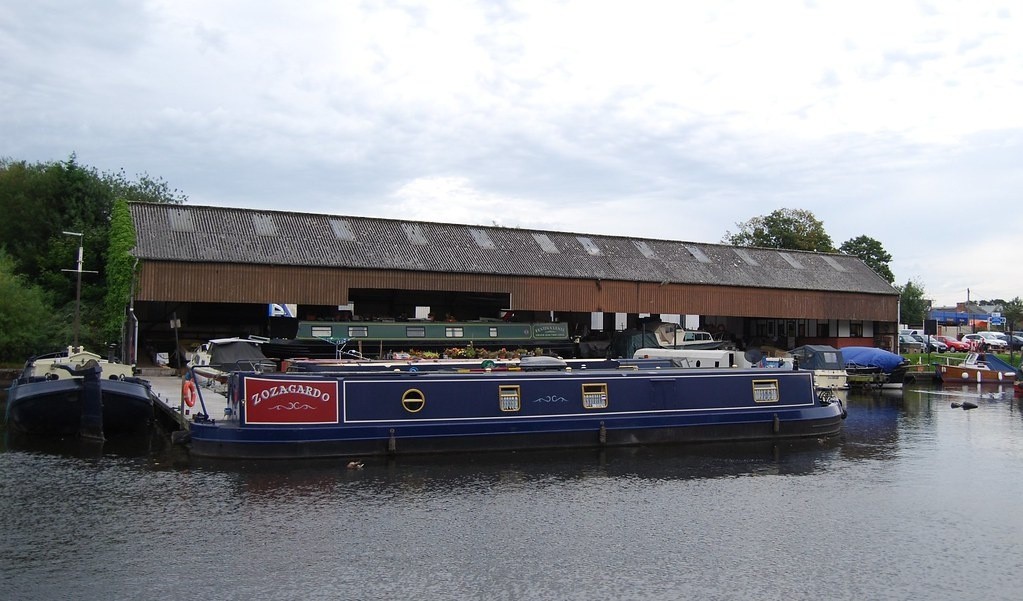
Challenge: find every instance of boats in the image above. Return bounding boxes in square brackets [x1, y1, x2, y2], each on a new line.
[617, 330, 726, 358]
[837, 346, 907, 387]
[188, 337, 279, 400]
[189, 353, 849, 455]
[787, 345, 851, 392]
[4, 345, 163, 448]
[933, 353, 1020, 385]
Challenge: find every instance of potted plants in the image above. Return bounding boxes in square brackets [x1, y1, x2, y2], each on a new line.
[488, 350, 497, 359]
[422, 351, 440, 359]
[512, 349, 526, 359]
[506, 351, 511, 359]
[535, 347, 543, 355]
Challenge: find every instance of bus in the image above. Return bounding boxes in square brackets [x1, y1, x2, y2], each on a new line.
[1005, 331, 1023, 336]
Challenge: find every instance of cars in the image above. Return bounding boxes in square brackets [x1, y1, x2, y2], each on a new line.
[997, 335, 1023, 351]
[912, 334, 948, 353]
[933, 336, 970, 353]
[899, 335, 927, 354]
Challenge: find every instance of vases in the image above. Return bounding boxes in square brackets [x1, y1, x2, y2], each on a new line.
[498, 354, 505, 359]
[452, 354, 489, 359]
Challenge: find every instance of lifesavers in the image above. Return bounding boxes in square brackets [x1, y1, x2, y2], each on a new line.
[184, 381, 196, 407]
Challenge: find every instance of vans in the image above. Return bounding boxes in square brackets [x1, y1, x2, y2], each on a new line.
[899, 329, 924, 336]
[977, 332, 1006, 340]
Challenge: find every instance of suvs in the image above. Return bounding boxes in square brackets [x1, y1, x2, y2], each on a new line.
[961, 334, 1008, 353]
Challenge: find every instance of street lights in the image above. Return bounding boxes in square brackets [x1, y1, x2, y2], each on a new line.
[62, 229, 99, 354]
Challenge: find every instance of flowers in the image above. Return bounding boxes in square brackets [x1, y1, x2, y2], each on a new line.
[410, 343, 508, 357]
